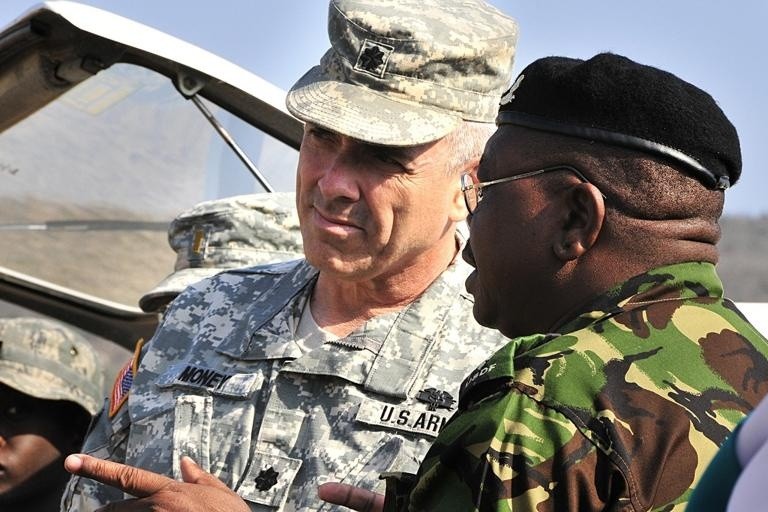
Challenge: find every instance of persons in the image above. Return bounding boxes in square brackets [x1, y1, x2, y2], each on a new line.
[64, 54, 768, 512]
[61, 0, 520, 511]
[2, 313, 110, 512]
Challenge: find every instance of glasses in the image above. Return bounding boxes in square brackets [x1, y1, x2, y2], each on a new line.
[462, 165, 608, 216]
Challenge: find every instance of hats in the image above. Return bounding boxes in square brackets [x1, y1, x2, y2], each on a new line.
[0, 318, 108, 418]
[496, 52, 742, 190]
[285, 0, 520, 149]
